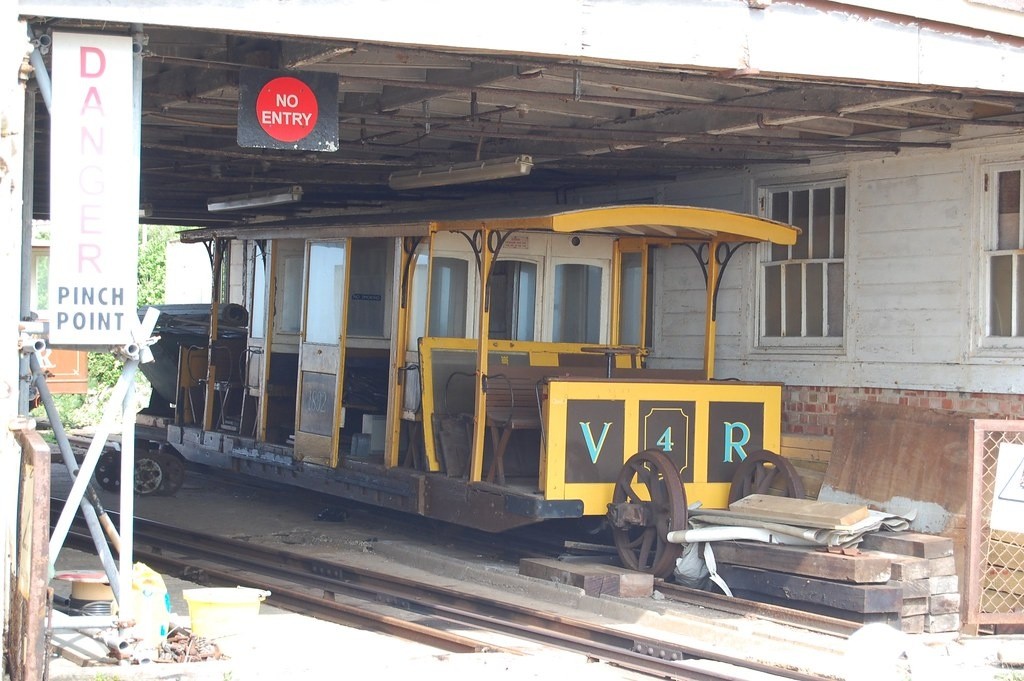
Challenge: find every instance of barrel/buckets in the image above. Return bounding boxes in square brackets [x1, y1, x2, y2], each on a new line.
[183, 586, 267, 640]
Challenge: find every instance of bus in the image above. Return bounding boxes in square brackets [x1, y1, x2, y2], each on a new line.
[94, 203, 803, 581]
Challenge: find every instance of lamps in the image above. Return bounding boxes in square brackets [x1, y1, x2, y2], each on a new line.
[388, 137, 533, 189]
[207, 162, 303, 211]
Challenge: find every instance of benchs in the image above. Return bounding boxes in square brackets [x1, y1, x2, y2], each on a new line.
[187, 338, 247, 430]
[444, 368, 740, 482]
[269, 385, 297, 398]
[342, 396, 386, 413]
